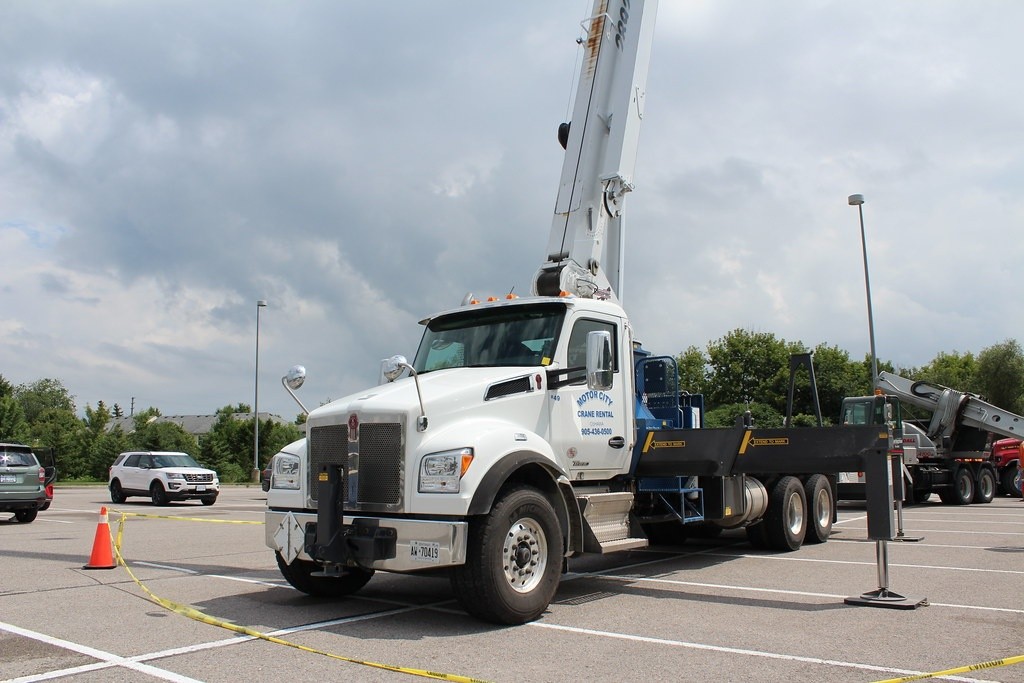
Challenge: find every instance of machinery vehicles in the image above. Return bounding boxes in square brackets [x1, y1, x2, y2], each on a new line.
[262, 1, 843, 628]
[839, 369, 1024, 507]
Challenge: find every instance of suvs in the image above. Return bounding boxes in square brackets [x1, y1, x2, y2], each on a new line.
[0, 443, 57, 522]
[107, 451, 220, 507]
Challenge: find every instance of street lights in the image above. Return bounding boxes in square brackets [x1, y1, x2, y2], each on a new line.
[252, 300, 267, 485]
[846, 192, 882, 394]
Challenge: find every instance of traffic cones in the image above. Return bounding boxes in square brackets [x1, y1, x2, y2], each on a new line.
[82, 507, 120, 570]
